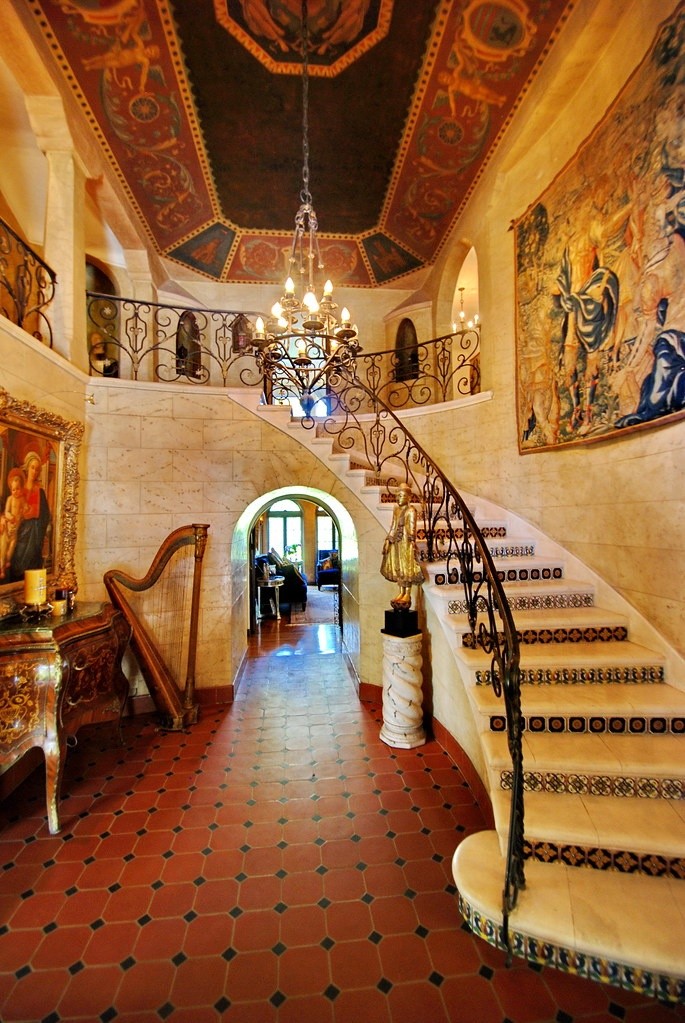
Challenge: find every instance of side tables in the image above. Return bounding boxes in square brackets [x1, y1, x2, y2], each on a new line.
[256, 575, 286, 622]
[0, 601, 133, 836]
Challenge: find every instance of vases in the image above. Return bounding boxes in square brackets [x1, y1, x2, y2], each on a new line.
[289, 553, 297, 563]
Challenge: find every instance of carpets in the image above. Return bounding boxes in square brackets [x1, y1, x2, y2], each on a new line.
[285, 587, 340, 627]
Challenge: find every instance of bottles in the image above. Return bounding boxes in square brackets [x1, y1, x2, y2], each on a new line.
[263, 562, 269, 580]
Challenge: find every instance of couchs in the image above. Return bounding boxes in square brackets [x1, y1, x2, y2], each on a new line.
[315, 549, 342, 591]
[255, 553, 308, 611]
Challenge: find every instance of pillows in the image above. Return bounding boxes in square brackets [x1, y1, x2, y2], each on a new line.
[280, 564, 305, 584]
[271, 547, 283, 563]
[319, 556, 333, 571]
[267, 551, 282, 569]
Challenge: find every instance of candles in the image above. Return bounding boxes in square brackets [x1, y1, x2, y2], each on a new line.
[22, 567, 47, 605]
[50, 600, 67, 616]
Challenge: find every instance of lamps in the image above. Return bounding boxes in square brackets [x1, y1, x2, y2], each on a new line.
[248, 205, 362, 424]
[447, 308, 482, 338]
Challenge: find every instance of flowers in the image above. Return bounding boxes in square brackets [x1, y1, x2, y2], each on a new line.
[284, 543, 302, 555]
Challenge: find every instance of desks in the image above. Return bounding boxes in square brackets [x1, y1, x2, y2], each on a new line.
[291, 560, 305, 574]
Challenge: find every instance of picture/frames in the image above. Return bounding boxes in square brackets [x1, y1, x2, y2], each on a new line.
[0, 384, 84, 624]
